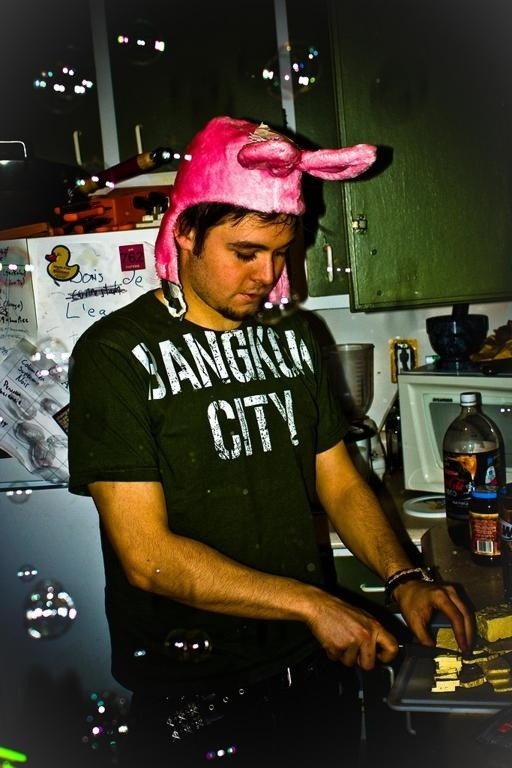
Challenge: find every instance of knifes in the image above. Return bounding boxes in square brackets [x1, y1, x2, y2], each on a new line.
[397, 640, 462, 661]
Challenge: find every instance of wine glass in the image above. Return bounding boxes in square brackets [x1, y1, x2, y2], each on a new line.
[423, 314, 489, 374]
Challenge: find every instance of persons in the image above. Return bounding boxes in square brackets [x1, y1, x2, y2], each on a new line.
[64, 113, 474, 766]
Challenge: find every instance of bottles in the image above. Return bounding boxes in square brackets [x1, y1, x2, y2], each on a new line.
[1, 153, 155, 229]
[442, 394, 505, 548]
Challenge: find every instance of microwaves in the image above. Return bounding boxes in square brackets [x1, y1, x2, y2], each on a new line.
[397, 359, 512, 494]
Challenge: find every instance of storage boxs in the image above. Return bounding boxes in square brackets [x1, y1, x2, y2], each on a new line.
[1, 229, 163, 367]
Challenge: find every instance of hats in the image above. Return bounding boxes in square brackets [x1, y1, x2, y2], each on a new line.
[153, 113, 379, 321]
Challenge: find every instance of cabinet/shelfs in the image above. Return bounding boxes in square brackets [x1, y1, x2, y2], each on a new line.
[285, 1, 510, 312]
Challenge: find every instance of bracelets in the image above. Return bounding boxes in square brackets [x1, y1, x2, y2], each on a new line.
[382, 563, 438, 605]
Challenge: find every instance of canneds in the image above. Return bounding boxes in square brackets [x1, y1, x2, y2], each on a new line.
[498, 483, 512, 556]
[468, 486, 502, 566]
